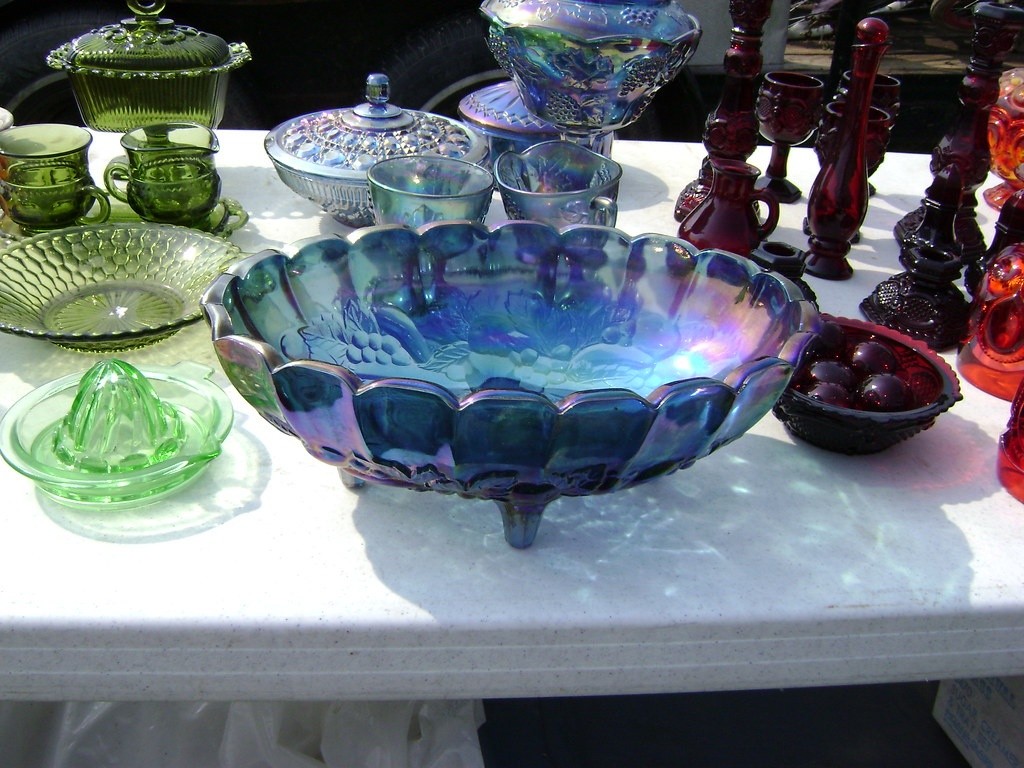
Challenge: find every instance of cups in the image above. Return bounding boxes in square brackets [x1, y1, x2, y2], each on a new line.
[0, 120, 220, 235]
[367, 154, 495, 226]
[494, 139, 623, 231]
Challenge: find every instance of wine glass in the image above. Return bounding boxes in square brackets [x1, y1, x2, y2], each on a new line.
[837, 72, 901, 195]
[803, 102, 891, 243]
[754, 72, 825, 202]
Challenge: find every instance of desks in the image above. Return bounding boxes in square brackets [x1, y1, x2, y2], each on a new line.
[1, 126, 1024, 702]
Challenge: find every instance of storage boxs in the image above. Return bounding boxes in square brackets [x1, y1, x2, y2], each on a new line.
[931, 676, 1024, 768]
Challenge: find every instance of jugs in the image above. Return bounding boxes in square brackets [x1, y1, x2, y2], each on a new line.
[676, 156, 780, 257]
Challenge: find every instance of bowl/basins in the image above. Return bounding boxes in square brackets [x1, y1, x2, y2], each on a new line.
[458, 79, 614, 188]
[263, 74, 489, 228]
[0, 221, 251, 353]
[767, 312, 962, 455]
[200, 219, 819, 548]
